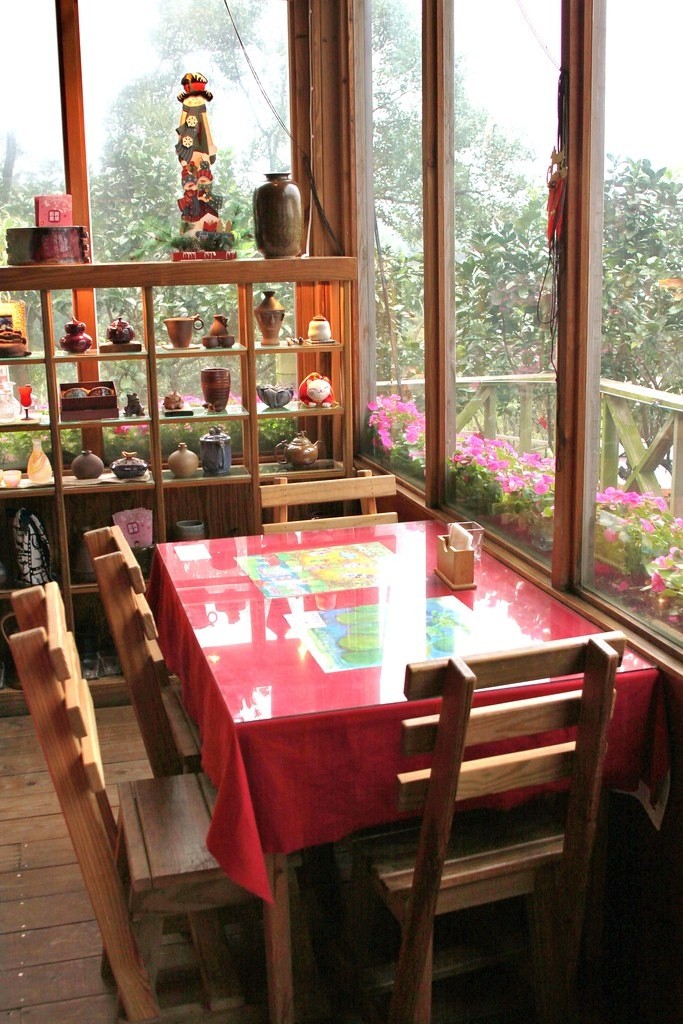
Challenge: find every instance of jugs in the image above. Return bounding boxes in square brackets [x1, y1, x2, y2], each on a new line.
[162, 314, 204, 348]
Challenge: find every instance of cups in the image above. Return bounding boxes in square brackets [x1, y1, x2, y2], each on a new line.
[176, 519, 204, 542]
[201, 368, 230, 412]
[3, 470, 21, 488]
[199, 426, 231, 475]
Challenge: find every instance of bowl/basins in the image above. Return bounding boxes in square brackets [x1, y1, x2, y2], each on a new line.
[257, 384, 294, 409]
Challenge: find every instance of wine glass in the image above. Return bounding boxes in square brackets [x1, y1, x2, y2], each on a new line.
[17, 386, 32, 420]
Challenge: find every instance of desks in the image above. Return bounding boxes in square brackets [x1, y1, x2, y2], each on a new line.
[146, 520, 658, 1024]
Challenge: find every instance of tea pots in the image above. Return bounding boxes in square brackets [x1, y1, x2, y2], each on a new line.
[274, 431, 324, 469]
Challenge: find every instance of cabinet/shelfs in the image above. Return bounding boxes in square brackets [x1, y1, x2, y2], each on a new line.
[1, 255, 360, 718]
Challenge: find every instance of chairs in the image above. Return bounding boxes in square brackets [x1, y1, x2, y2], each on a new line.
[85, 527, 203, 778]
[8, 583, 308, 1024]
[335, 629, 625, 1023]
[259, 471, 399, 535]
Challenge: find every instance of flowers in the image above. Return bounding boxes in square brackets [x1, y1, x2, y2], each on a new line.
[368, 394, 682, 630]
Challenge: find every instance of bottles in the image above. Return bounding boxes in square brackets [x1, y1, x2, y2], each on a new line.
[0, 382, 20, 422]
[71, 450, 104, 480]
[110, 452, 149, 480]
[307, 314, 331, 341]
[210, 315, 228, 336]
[167, 443, 198, 478]
[27, 440, 52, 485]
